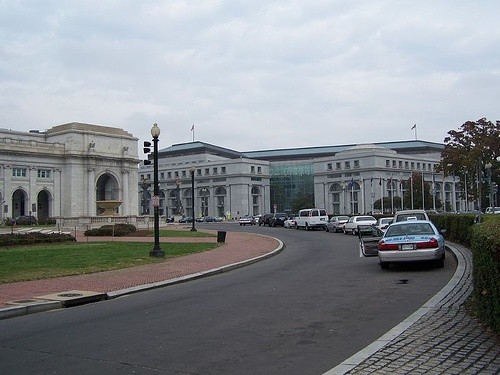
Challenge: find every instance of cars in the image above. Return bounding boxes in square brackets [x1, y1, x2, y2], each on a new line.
[485, 206, 500, 215]
[8, 215, 36, 226]
[357, 220, 446, 270]
[342, 215, 394, 235]
[325, 216, 351, 232]
[239, 212, 298, 228]
[167, 212, 222, 222]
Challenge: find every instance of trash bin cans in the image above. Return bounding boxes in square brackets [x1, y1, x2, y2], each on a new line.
[217, 230, 227, 243]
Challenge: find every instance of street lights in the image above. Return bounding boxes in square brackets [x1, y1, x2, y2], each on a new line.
[150, 123, 164, 257]
[190, 164, 198, 231]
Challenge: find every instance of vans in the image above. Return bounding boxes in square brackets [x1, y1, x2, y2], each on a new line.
[392, 210, 430, 223]
[295, 208, 329, 231]
